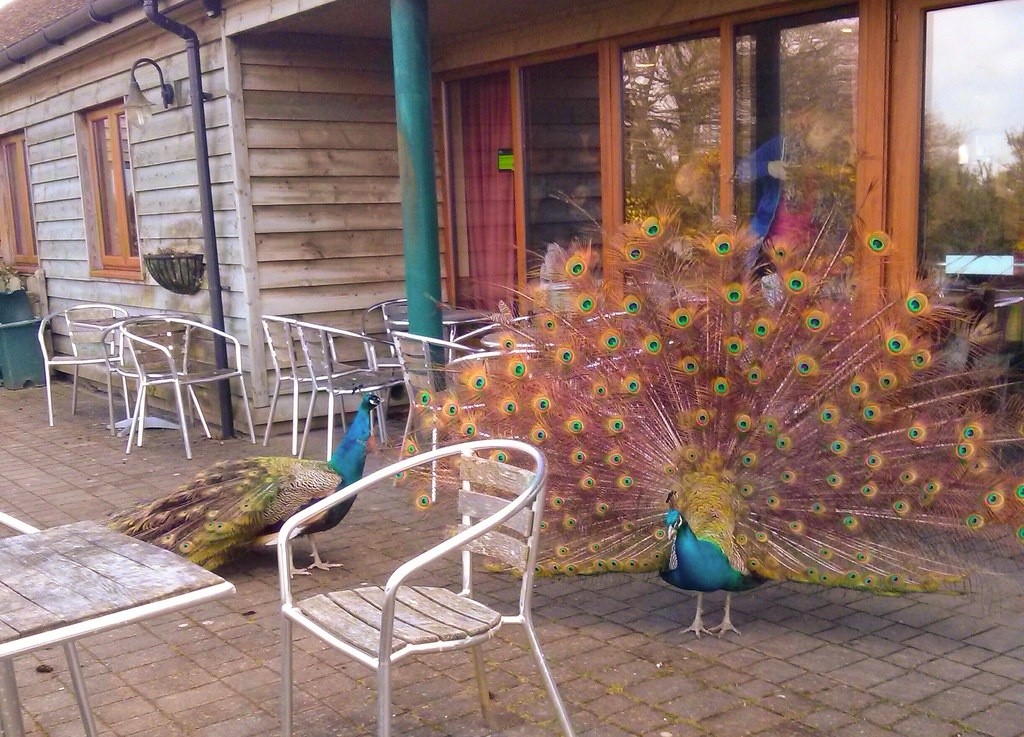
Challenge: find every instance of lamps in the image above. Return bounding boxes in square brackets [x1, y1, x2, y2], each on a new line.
[121, 57, 176, 126]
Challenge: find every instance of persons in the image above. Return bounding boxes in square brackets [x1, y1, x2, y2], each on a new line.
[734, 108, 854, 283]
[531, 133, 718, 294]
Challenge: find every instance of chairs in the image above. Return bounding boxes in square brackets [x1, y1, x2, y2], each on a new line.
[32, 304, 132, 426]
[261, 297, 711, 504]
[277, 439, 576, 737]
[99, 315, 258, 460]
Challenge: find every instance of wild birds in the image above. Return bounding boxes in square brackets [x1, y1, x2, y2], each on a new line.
[397, 149, 1024, 639]
[96, 385, 387, 580]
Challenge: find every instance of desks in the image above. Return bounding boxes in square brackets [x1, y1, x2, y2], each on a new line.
[0, 520, 238, 737]
[479, 327, 576, 350]
[71, 315, 203, 438]
[386, 306, 497, 350]
